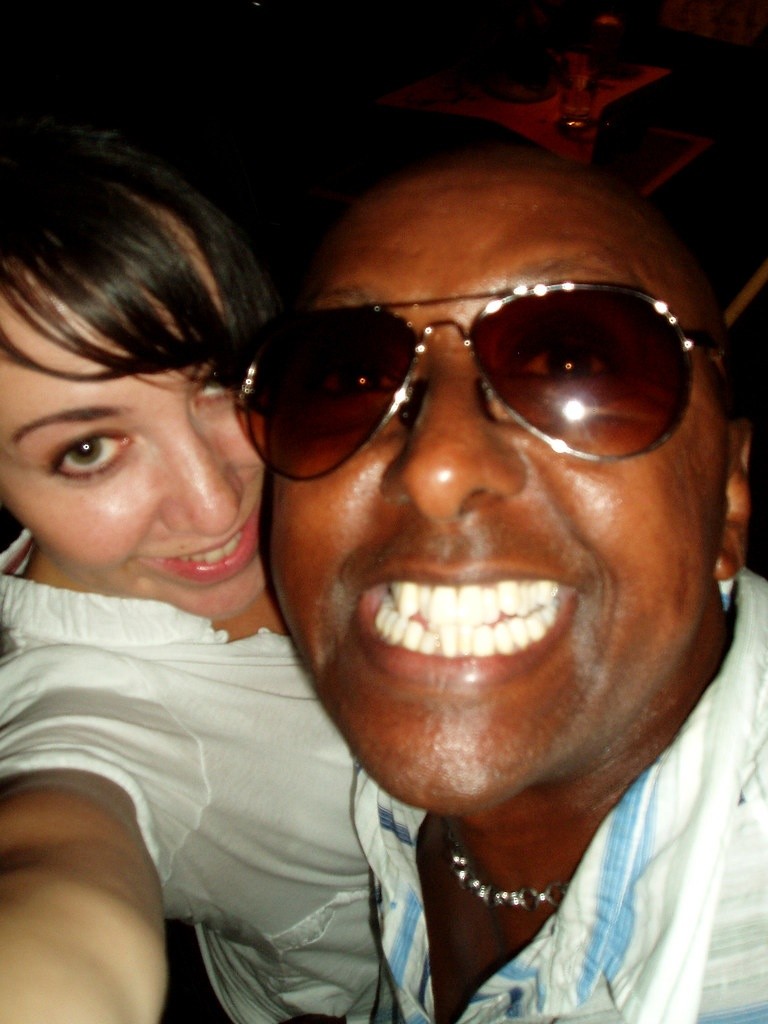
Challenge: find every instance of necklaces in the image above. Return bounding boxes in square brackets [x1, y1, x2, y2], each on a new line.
[443, 814, 572, 912]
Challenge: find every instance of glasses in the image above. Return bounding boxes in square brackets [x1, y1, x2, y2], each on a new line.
[235, 281, 738, 479]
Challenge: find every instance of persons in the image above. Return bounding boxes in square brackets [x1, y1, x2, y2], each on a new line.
[0, 120, 408, 1024]
[269, 142, 768, 1024]
